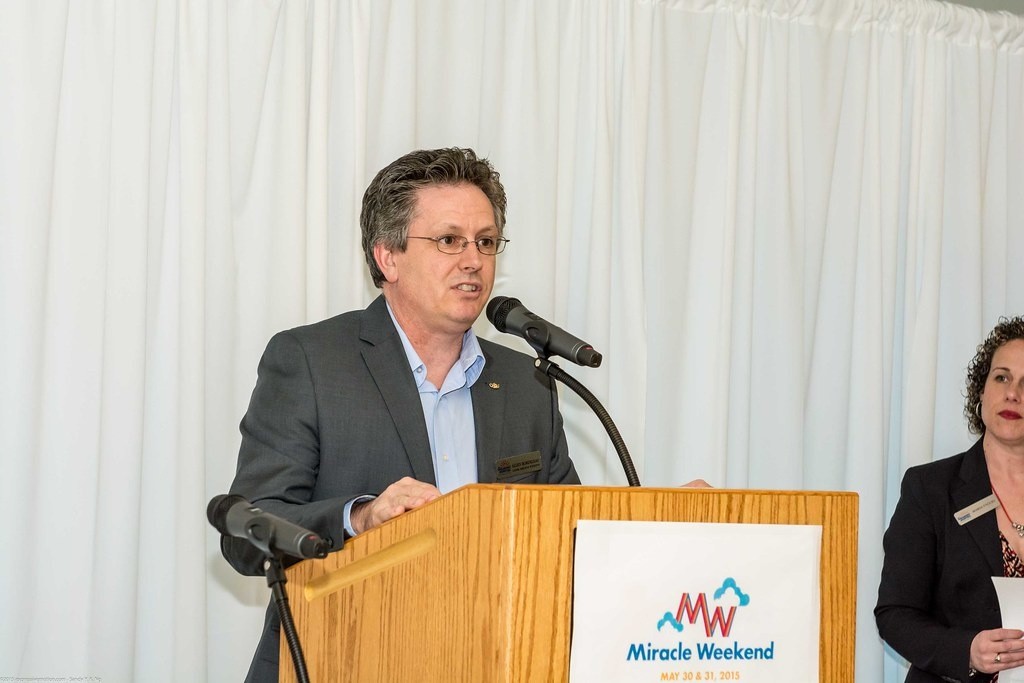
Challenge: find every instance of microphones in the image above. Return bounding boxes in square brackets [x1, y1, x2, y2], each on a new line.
[486, 295, 603, 368]
[207, 494, 328, 560]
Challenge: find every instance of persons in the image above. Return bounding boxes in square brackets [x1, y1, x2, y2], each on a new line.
[873, 314, 1024, 683]
[220, 147, 717, 683]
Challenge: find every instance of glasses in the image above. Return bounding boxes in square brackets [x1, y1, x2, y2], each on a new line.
[406, 233, 510, 256]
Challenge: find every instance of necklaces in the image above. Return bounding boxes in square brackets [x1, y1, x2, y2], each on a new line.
[990, 480, 1024, 537]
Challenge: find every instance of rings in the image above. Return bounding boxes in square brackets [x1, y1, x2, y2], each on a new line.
[995, 652, 1001, 664]
